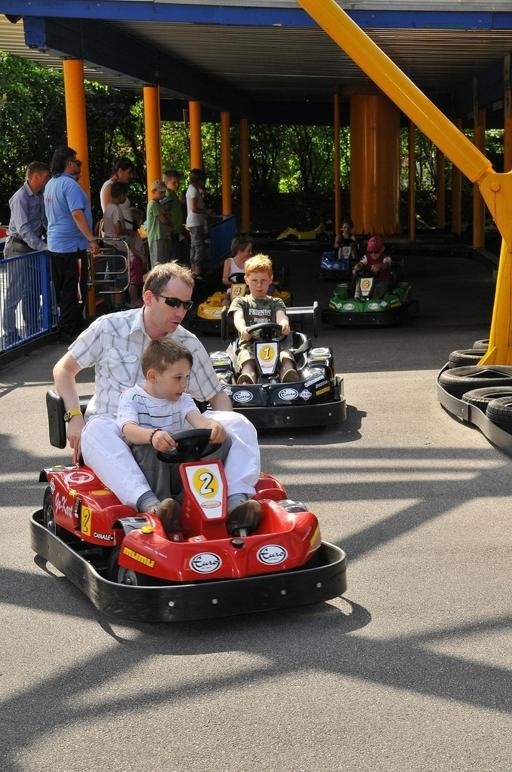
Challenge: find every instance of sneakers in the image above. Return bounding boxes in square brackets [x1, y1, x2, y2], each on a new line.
[113, 303, 130, 313]
[149, 499, 181, 531]
[225, 499, 262, 534]
[236, 373, 254, 384]
[282, 369, 299, 382]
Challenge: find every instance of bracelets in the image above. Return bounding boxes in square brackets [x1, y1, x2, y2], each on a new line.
[149, 428, 163, 448]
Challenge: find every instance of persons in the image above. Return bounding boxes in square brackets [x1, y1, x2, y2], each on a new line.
[1, 145, 93, 349]
[224, 252, 300, 385]
[347, 235, 394, 300]
[222, 235, 275, 306]
[51, 258, 264, 538]
[335, 219, 359, 260]
[116, 334, 231, 502]
[101, 157, 210, 310]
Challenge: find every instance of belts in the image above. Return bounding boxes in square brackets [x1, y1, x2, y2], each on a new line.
[11, 237, 28, 246]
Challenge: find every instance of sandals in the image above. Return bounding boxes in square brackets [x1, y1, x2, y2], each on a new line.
[192, 273, 208, 282]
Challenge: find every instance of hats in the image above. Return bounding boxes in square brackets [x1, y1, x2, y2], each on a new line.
[150, 180, 167, 192]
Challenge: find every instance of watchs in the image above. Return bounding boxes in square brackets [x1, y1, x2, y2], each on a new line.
[63, 409, 83, 422]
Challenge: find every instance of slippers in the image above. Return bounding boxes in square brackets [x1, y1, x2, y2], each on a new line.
[125, 299, 143, 307]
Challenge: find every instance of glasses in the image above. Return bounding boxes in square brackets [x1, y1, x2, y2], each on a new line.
[160, 294, 193, 311]
[71, 160, 81, 166]
[369, 251, 377, 254]
